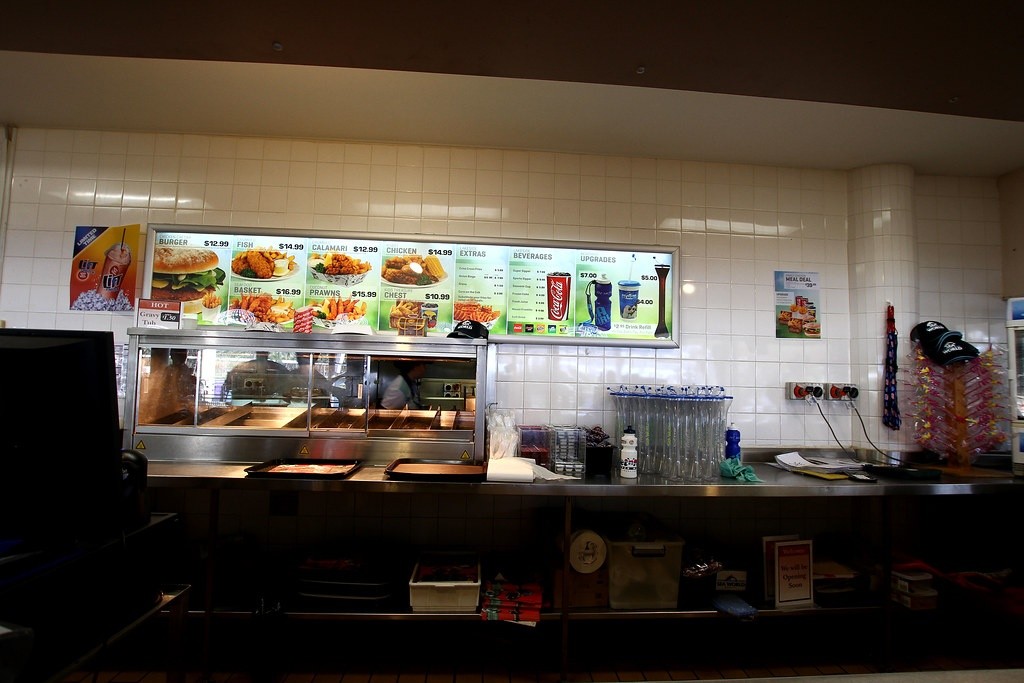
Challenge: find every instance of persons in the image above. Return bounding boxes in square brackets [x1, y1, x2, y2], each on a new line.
[153, 343, 341, 410]
[380, 360, 428, 410]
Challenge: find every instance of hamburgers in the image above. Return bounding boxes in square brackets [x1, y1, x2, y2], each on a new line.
[150, 248, 226, 301]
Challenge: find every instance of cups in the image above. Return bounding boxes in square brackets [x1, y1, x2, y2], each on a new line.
[796, 296, 816, 318]
[547, 276, 571, 321]
[96, 254, 131, 299]
[421, 305, 438, 329]
[619, 284, 640, 318]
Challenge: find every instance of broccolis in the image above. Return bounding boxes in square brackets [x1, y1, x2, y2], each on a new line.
[311, 263, 325, 273]
[241, 269, 255, 278]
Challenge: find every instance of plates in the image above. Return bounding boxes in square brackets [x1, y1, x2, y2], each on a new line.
[231, 262, 300, 280]
[381, 273, 449, 287]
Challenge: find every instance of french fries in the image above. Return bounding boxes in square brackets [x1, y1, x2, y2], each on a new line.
[202, 292, 221, 309]
[266, 295, 295, 323]
[234, 246, 296, 271]
[349, 302, 367, 321]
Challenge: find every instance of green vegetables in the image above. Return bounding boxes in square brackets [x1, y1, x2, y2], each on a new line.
[416, 273, 432, 285]
[315, 310, 326, 319]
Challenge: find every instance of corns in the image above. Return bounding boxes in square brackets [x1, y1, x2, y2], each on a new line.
[423, 256, 444, 278]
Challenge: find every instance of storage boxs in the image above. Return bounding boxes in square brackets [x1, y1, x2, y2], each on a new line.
[552, 523, 685, 612]
[409, 551, 482, 614]
[891, 568, 938, 611]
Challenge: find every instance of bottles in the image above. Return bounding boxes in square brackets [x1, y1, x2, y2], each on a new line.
[595, 274, 612, 330]
[726, 422, 741, 462]
[621, 425, 637, 478]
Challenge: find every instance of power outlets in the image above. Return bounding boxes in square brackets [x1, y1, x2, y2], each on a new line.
[786, 381, 825, 402]
[823, 384, 859, 401]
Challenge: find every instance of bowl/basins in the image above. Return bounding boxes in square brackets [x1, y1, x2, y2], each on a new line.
[455, 316, 501, 331]
